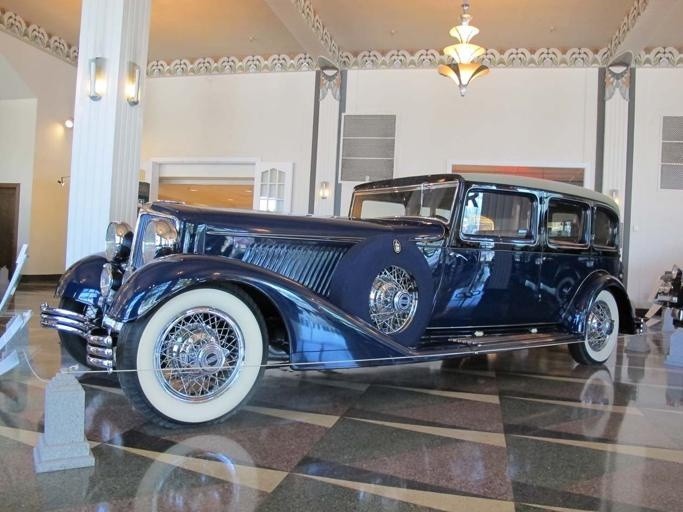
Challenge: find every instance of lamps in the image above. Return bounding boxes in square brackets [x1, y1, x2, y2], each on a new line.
[436, 0, 493, 95]
[87, 56, 108, 101]
[123, 61, 142, 106]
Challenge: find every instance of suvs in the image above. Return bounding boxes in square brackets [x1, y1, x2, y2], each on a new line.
[36, 163, 636, 433]
[76, 365, 636, 512]
